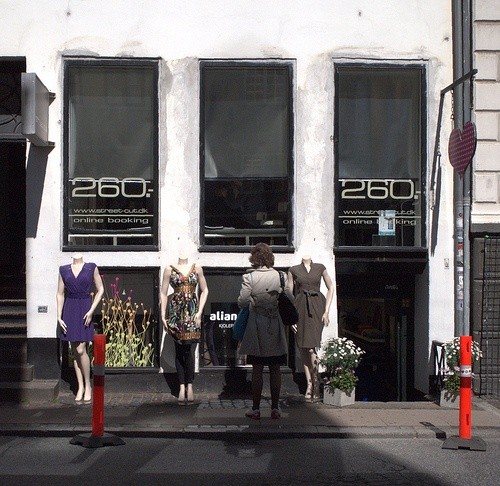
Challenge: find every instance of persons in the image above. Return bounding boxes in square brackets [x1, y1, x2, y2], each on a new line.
[358, 357, 382, 401]
[56, 253, 104, 404]
[288, 255, 334, 399]
[238, 243, 288, 419]
[160, 255, 208, 405]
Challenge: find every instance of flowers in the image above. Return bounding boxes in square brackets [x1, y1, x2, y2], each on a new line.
[442, 337, 483, 394]
[313, 337, 366, 394]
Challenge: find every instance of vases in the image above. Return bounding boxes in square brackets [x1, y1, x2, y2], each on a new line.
[323, 386, 356, 406]
[440, 390, 461, 409]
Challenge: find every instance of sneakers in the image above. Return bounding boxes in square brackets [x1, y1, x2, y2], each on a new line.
[270, 408, 281, 418]
[246, 406, 260, 419]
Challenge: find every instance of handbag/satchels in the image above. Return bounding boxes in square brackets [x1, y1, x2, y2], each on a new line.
[278, 269, 300, 327]
